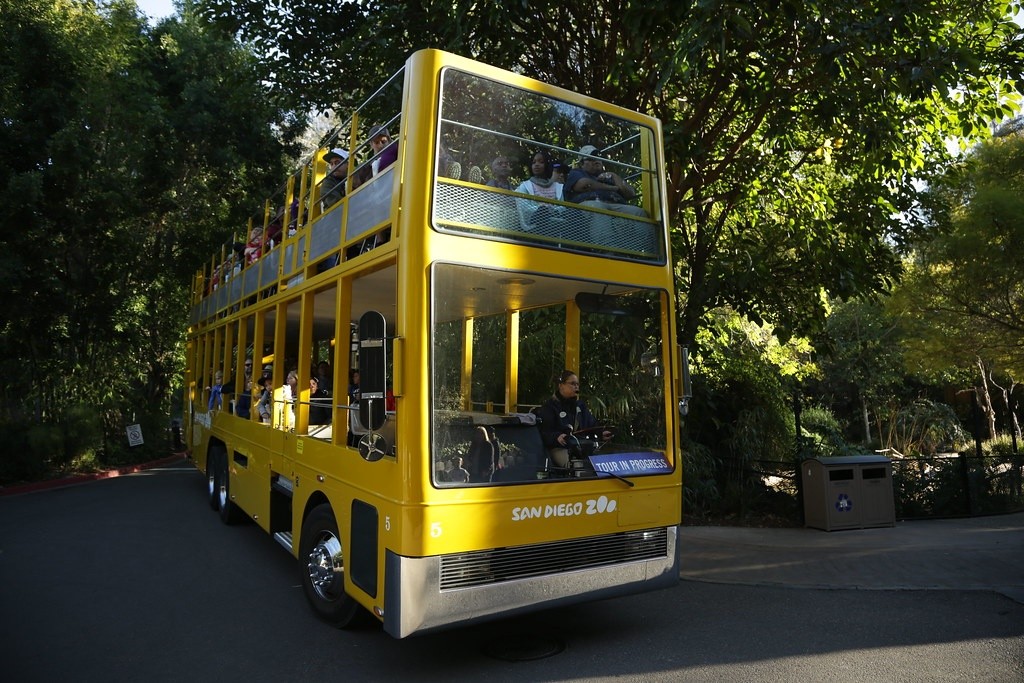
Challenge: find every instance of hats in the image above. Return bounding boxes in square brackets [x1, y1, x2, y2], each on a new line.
[257, 370, 272, 386]
[575, 145, 599, 164]
[368, 125, 391, 141]
[322, 149, 349, 162]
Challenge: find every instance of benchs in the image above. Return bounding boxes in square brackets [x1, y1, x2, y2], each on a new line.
[285, 398, 352, 441]
[437, 197, 572, 237]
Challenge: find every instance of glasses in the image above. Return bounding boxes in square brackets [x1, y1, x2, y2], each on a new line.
[563, 381, 580, 386]
[581, 157, 594, 161]
[245, 363, 253, 367]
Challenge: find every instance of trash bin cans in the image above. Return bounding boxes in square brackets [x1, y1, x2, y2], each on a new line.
[800, 455, 896, 531]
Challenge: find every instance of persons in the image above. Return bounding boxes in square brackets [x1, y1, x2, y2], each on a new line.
[350, 369, 360, 402]
[514, 151, 567, 241]
[208, 370, 223, 418]
[235, 376, 252, 419]
[196, 366, 214, 391]
[447, 457, 471, 483]
[220, 359, 253, 393]
[309, 376, 329, 426]
[261, 365, 272, 376]
[286, 370, 298, 409]
[311, 361, 334, 390]
[256, 373, 273, 423]
[563, 144, 656, 260]
[202, 126, 398, 293]
[484, 425, 501, 482]
[467, 426, 494, 483]
[484, 156, 517, 192]
[536, 369, 614, 483]
[551, 164, 570, 183]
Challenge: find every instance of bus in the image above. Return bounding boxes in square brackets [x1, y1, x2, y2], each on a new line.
[183, 50, 685, 640]
